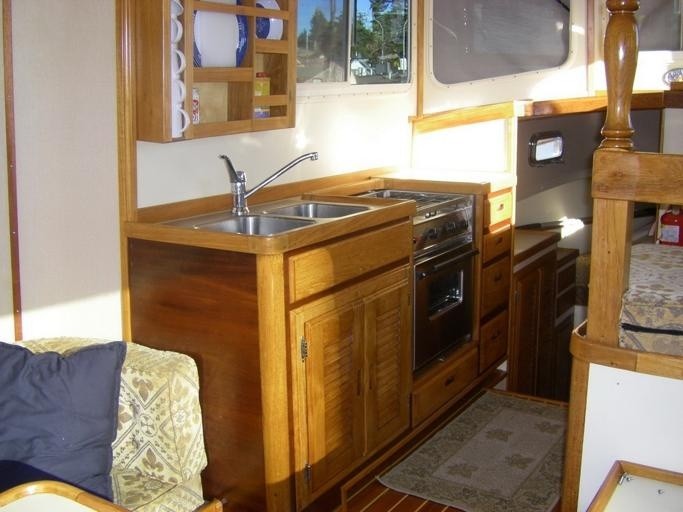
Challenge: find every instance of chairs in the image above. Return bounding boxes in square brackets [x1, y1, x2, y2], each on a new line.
[0, 338, 208, 510]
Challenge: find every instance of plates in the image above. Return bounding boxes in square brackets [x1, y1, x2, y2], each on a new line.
[194, 0, 249, 68]
[255, 1, 283, 40]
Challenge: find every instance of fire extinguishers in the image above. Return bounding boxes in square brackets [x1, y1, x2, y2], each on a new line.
[659, 204, 683, 247]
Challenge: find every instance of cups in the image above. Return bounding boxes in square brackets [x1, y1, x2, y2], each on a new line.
[169, 0, 190, 139]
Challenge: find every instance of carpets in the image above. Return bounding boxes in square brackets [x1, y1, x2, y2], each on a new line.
[374, 390, 568, 510]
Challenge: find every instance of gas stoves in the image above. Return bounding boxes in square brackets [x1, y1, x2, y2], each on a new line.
[349, 188, 467, 212]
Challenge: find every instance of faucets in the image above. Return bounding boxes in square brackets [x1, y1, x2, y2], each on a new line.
[219, 152, 318, 216]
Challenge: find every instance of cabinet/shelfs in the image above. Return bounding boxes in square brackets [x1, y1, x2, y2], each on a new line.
[127, 220, 411, 511]
[131, 0, 298, 144]
[509, 230, 562, 386]
[478, 187, 515, 391]
[554, 248, 580, 334]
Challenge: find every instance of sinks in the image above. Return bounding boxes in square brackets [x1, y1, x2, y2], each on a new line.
[264, 202, 370, 218]
[195, 216, 316, 235]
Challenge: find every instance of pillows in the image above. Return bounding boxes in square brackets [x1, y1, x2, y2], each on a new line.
[0, 339, 126, 500]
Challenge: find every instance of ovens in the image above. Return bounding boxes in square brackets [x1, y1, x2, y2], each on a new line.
[412, 196, 478, 373]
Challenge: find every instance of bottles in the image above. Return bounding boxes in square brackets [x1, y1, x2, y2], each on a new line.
[254, 72, 271, 118]
[192, 87, 199, 124]
